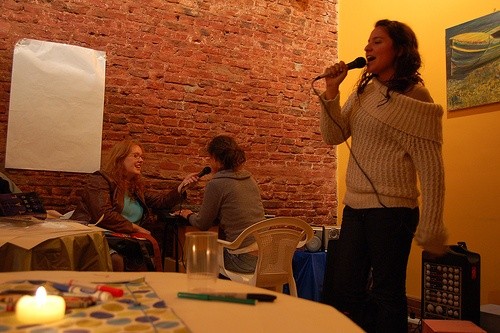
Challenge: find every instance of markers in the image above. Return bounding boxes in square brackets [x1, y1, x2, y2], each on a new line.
[0, 279, 125, 311]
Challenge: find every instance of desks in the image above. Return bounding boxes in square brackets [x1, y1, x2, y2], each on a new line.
[0, 270, 367, 333]
[283, 251, 326, 302]
[0, 227, 113, 273]
[160, 217, 184, 273]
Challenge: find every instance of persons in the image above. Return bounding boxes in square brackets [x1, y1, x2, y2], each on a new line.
[175, 136, 264, 279]
[87, 140, 200, 271]
[318, 18, 447, 333]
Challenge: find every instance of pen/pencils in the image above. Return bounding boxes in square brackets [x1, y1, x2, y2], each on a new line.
[177, 291, 277, 305]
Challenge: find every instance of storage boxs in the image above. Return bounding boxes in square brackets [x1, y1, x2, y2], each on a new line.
[422, 319, 487, 333]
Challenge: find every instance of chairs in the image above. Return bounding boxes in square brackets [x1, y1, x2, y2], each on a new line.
[216, 216, 314, 297]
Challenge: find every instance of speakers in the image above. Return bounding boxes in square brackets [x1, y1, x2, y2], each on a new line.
[304, 226, 323, 252]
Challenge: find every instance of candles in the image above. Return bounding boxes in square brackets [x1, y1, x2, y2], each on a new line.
[16, 286, 66, 323]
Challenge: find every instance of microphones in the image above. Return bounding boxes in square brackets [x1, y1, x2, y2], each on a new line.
[315, 57, 367, 80]
[182, 166, 212, 188]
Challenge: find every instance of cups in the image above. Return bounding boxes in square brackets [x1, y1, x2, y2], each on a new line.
[184, 232, 218, 293]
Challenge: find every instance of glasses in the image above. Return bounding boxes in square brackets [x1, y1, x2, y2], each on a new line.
[125, 153, 144, 161]
